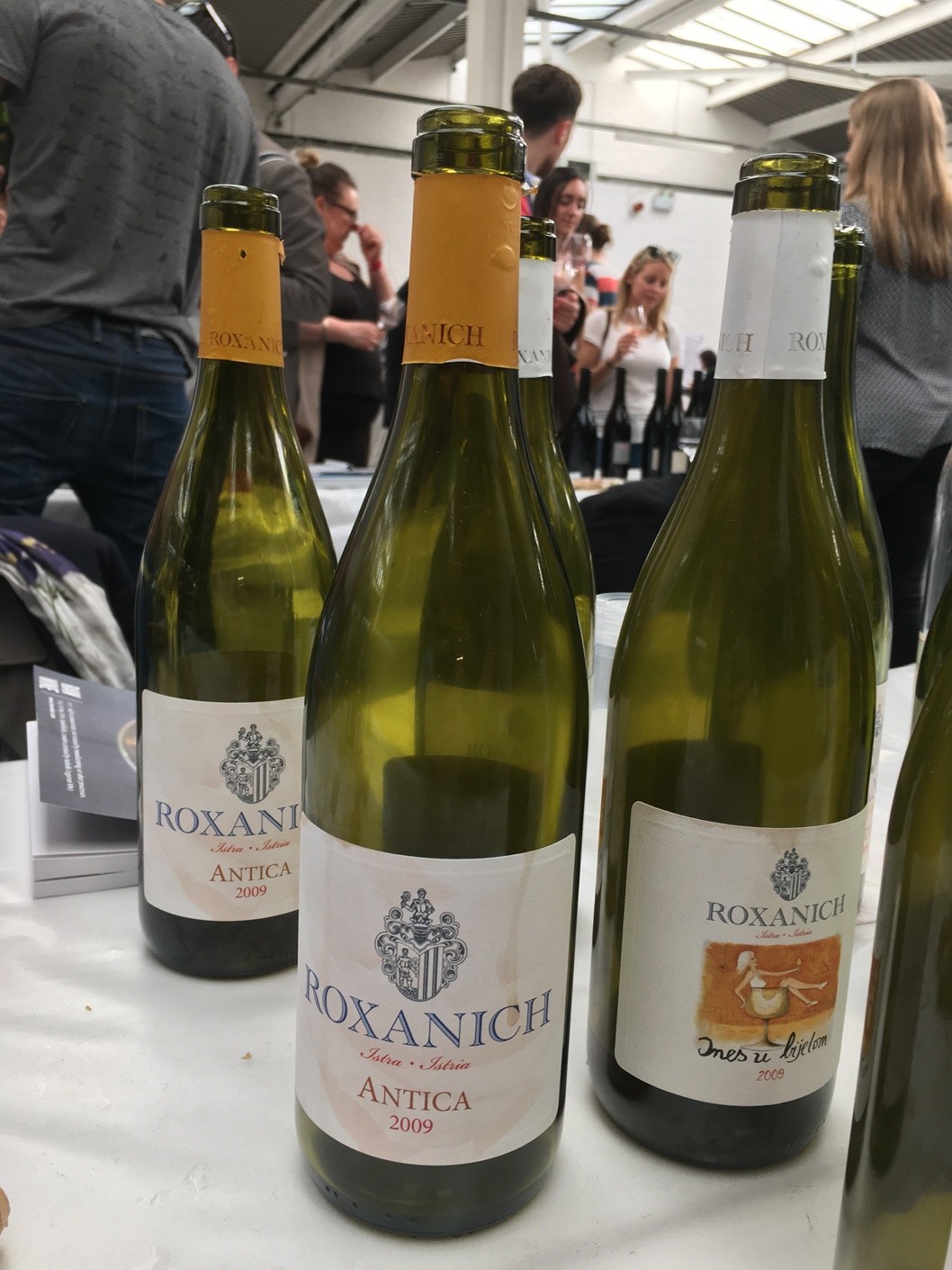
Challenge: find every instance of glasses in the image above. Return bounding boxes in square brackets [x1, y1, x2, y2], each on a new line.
[324, 196, 356, 225]
[173, 0, 236, 57]
[635, 247, 678, 266]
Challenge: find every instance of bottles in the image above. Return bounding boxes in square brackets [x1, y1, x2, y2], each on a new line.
[128, 180, 345, 982]
[286, 101, 599, 1240]
[559, 365, 715, 487]
[825, 581, 951, 1270]
[587, 146, 899, 1164]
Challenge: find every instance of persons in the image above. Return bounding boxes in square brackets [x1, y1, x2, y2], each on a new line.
[0, 0, 257, 677]
[178, 9, 717, 489]
[837, 78, 952, 669]
